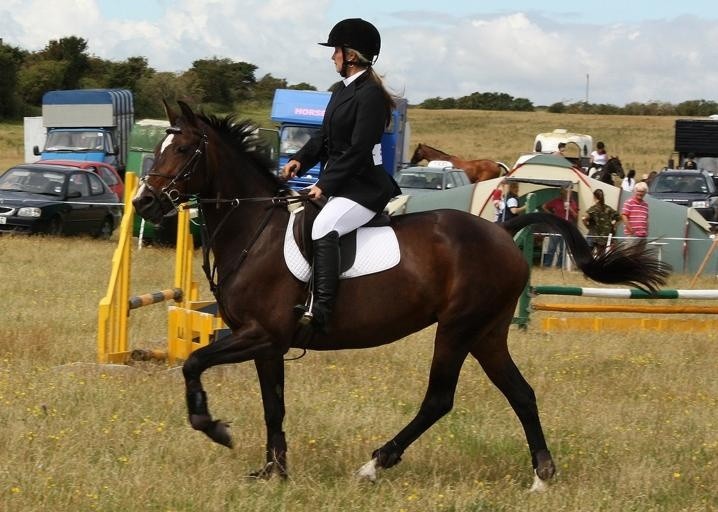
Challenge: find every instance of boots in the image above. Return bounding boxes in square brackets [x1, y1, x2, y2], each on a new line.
[292, 228, 341, 330]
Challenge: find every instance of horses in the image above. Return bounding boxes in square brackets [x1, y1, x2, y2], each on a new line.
[410, 143, 509, 184]
[132, 98, 672, 492]
[582, 155, 625, 186]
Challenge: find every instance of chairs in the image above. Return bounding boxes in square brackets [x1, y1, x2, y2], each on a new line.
[658, 179, 704, 194]
[53, 134, 73, 146]
[401, 175, 441, 189]
[14, 175, 62, 194]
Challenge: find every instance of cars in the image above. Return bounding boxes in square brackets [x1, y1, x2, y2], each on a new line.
[390, 159, 472, 197]
[648, 168, 718, 231]
[1, 164, 122, 241]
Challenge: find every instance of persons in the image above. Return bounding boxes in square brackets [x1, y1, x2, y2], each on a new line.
[554, 142, 568, 156]
[684, 153, 697, 169]
[541, 187, 578, 268]
[279, 15, 402, 331]
[584, 186, 620, 262]
[621, 181, 650, 260]
[587, 142, 607, 178]
[619, 169, 635, 192]
[496, 181, 528, 228]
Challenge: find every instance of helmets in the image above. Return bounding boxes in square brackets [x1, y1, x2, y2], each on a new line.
[317, 17, 382, 56]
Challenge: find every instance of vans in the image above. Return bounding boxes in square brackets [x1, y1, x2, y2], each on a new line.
[33, 159, 124, 202]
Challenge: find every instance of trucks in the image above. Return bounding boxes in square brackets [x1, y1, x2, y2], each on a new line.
[666, 119, 718, 191]
[32, 88, 134, 182]
[269, 88, 411, 201]
